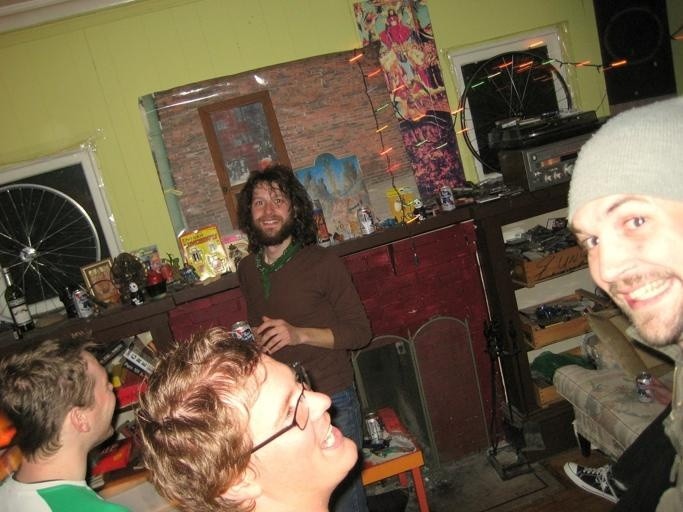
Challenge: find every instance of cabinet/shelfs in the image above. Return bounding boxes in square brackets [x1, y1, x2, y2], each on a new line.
[471, 182, 620, 459]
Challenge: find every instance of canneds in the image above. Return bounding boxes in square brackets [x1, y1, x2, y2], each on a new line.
[636, 372, 655, 403]
[71, 288, 95, 320]
[357, 206, 376, 236]
[231, 320, 255, 343]
[438, 185, 456, 212]
[364, 412, 384, 446]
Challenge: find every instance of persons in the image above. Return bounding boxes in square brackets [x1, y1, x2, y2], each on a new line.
[131, 326, 360, 512]
[566, 93, 681, 512]
[233, 163, 375, 512]
[0, 328, 129, 512]
[561, 373, 678, 512]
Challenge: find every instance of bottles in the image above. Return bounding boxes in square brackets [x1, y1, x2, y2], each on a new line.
[1, 267, 35, 333]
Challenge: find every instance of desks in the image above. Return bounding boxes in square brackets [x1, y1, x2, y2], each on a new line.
[360, 406, 430, 512]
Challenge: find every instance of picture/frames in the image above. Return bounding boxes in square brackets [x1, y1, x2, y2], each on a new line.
[79, 257, 119, 305]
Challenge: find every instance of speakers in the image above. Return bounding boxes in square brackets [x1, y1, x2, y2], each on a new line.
[592, 0, 677, 106]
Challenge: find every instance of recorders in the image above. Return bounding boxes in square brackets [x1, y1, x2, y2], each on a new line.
[498, 133, 594, 191]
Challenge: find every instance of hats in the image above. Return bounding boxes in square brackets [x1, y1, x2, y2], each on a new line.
[568, 97, 683, 230]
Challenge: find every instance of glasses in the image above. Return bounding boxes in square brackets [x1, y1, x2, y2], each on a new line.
[247, 363, 311, 453]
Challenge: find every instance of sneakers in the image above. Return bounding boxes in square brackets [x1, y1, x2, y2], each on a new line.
[564, 462, 620, 504]
